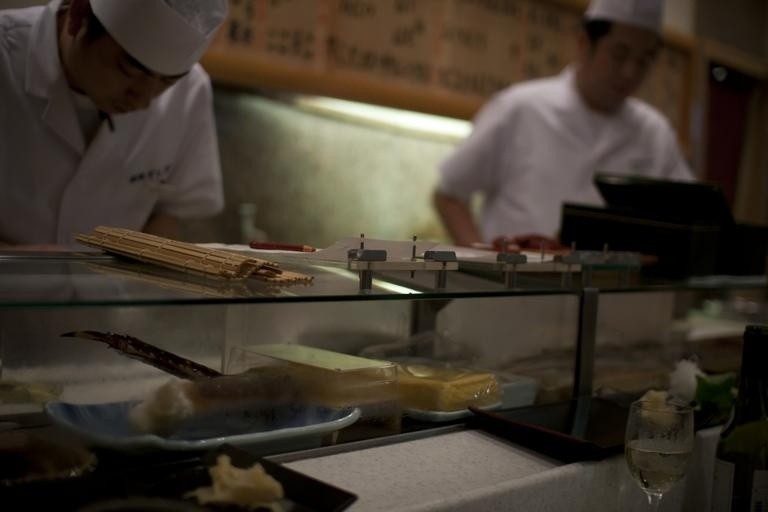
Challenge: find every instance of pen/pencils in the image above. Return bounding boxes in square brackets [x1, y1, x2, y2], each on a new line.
[249, 240, 315, 252]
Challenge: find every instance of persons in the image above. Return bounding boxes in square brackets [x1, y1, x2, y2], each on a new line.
[0, 0, 230, 375]
[428, 0, 699, 367]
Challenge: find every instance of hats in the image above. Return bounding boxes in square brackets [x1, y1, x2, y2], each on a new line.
[89, 3, 233, 77]
[585, 0, 667, 34]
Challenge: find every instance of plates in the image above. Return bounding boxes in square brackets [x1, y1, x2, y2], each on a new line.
[402, 400, 503, 422]
[43, 398, 364, 466]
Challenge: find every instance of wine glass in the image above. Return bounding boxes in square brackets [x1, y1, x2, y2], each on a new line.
[624, 399, 695, 512]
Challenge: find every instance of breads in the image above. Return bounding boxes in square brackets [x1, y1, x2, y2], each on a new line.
[132, 341, 500, 438]
